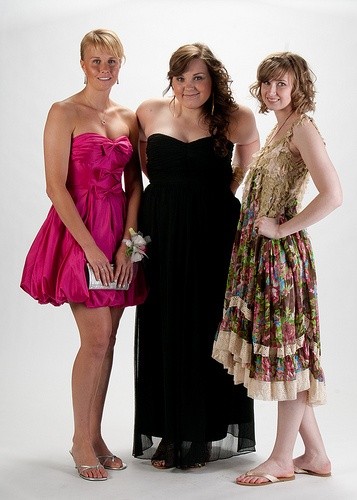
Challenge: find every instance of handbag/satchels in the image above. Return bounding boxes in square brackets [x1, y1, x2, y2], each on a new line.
[86, 263, 129, 290]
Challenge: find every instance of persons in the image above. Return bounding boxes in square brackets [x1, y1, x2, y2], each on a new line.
[131, 43, 260, 470]
[211, 51, 344, 486]
[19, 28, 146, 481]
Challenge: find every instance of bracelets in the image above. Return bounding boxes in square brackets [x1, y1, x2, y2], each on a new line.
[121, 228, 150, 263]
[231, 166, 246, 185]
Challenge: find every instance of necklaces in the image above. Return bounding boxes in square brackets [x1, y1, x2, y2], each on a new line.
[265, 109, 297, 146]
[82, 89, 109, 125]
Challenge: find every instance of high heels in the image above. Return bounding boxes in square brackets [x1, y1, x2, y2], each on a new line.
[176, 441, 205, 468]
[150, 438, 183, 468]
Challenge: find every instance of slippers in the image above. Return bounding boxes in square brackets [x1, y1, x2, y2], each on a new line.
[97, 454, 126, 470]
[292, 465, 331, 477]
[68, 444, 108, 481]
[236, 470, 295, 485]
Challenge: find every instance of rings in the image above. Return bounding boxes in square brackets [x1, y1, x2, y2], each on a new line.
[254, 227, 258, 231]
[99, 268, 105, 271]
